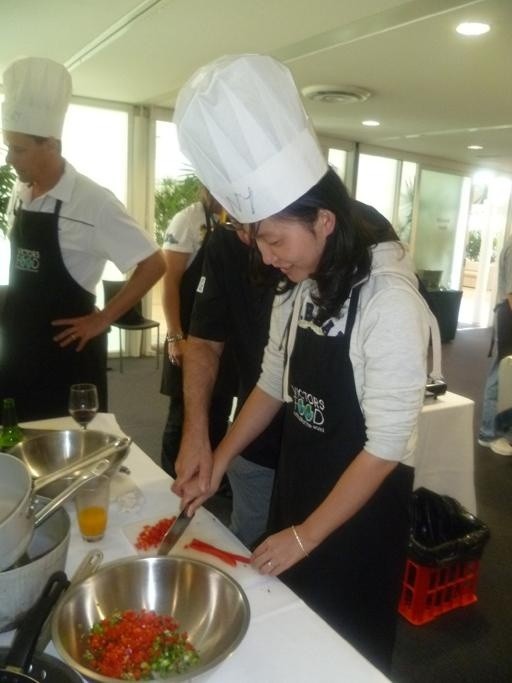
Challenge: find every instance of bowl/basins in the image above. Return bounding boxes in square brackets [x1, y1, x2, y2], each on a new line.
[8, 430, 130, 501]
[51, 555, 251, 683]
[1, 494, 71, 633]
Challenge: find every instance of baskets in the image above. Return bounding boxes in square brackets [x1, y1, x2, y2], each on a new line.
[398, 556, 477, 626]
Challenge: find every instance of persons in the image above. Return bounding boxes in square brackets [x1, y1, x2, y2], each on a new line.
[172, 167, 431, 675]
[159, 184, 237, 502]
[476, 233, 510, 460]
[170, 198, 404, 553]
[0, 131, 165, 417]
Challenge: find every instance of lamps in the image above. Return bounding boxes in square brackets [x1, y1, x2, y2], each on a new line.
[302, 83, 371, 106]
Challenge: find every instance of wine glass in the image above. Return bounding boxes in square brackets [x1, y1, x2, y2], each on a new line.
[69, 384, 99, 430]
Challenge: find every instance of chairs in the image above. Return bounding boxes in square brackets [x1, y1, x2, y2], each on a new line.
[101, 278, 162, 375]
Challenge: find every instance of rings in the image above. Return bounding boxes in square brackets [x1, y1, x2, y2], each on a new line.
[70, 334, 78, 342]
[266, 560, 275, 568]
[171, 360, 177, 363]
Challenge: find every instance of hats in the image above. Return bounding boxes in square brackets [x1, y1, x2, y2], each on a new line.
[172, 51, 330, 224]
[2, 57, 73, 139]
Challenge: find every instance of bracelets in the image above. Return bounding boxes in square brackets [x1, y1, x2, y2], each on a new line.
[167, 332, 184, 342]
[290, 522, 315, 563]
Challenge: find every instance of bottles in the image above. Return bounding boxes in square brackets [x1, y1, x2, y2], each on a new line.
[0, 398, 23, 452]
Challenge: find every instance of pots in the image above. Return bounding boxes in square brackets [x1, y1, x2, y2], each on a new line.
[0, 549, 104, 683]
[0, 436, 133, 572]
[0, 570, 67, 683]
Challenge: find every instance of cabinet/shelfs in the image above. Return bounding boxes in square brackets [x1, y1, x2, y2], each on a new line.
[424, 289, 463, 343]
[410, 390, 477, 518]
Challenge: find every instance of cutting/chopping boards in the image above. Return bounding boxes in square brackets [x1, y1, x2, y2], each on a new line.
[122, 512, 257, 582]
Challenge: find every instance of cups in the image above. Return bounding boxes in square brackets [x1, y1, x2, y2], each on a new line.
[74, 474, 111, 542]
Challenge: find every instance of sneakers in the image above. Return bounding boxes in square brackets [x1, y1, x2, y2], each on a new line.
[478, 437, 512, 456]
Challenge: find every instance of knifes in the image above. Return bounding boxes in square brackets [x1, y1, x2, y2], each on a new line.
[156, 497, 195, 555]
